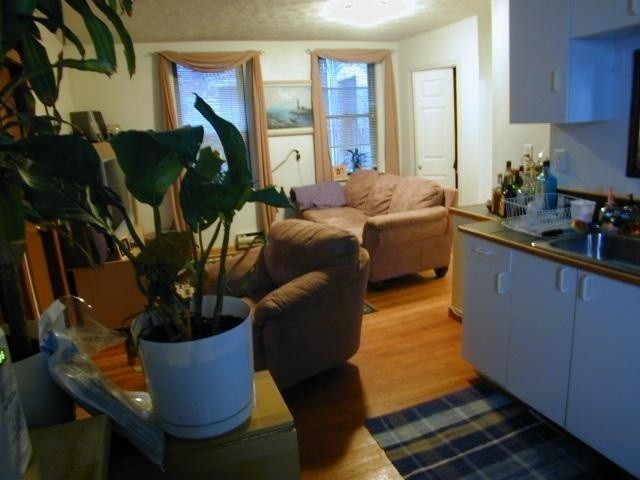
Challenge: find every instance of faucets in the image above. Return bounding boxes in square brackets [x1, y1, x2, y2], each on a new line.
[600, 205, 638, 231]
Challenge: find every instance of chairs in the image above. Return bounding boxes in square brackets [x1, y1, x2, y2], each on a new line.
[227, 215, 371, 392]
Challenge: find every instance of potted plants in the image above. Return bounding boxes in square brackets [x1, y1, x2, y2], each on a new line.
[3, 92, 296, 443]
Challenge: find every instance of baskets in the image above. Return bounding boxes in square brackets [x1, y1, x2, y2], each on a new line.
[502, 192, 589, 234]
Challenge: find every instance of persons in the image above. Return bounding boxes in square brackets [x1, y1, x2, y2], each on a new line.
[351, 148, 362, 171]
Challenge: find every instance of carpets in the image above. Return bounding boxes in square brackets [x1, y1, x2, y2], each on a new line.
[361, 379, 638, 479]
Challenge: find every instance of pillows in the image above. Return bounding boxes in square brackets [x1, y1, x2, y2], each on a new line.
[290, 181, 348, 209]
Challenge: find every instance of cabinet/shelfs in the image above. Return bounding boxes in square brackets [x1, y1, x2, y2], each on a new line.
[569, 0, 640, 35]
[508, 1, 611, 124]
[459, 229, 512, 393]
[512, 248, 640, 475]
[131, 370, 301, 480]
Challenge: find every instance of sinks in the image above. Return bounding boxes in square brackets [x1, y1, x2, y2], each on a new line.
[531, 231, 639, 274]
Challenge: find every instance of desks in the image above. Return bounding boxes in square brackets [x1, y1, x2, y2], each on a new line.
[20, 414, 112, 480]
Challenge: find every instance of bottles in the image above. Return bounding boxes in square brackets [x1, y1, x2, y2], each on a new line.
[491, 161, 557, 217]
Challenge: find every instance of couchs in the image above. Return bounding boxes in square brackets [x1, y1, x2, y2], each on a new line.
[300, 168, 458, 290]
